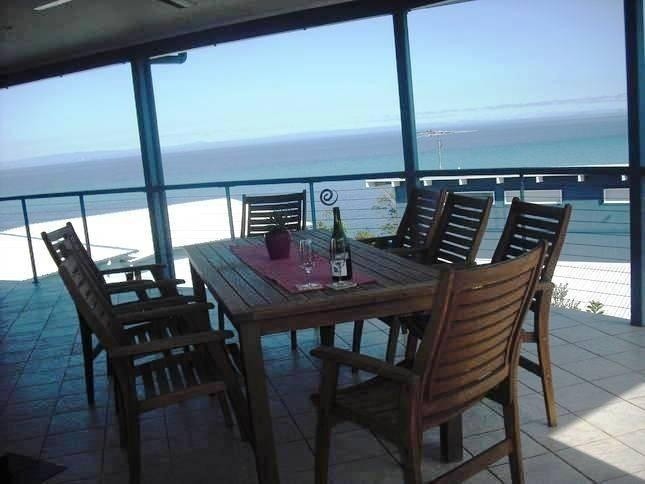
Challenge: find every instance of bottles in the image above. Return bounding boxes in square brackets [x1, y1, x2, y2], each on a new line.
[329, 207, 352, 282]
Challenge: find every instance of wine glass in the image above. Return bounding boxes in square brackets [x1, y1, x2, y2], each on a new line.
[298, 239, 320, 290]
[331, 238, 349, 287]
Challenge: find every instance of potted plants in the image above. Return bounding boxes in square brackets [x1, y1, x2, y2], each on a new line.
[264, 207, 292, 260]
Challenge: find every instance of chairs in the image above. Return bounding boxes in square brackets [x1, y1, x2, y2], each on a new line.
[41, 222, 253, 484]
[309, 239, 547, 484]
[218, 190, 306, 352]
[352, 190, 571, 427]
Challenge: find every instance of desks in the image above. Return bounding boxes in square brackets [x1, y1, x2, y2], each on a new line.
[183, 229, 439, 484]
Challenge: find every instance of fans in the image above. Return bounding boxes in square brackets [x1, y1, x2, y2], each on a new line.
[33, 0, 191, 11]
